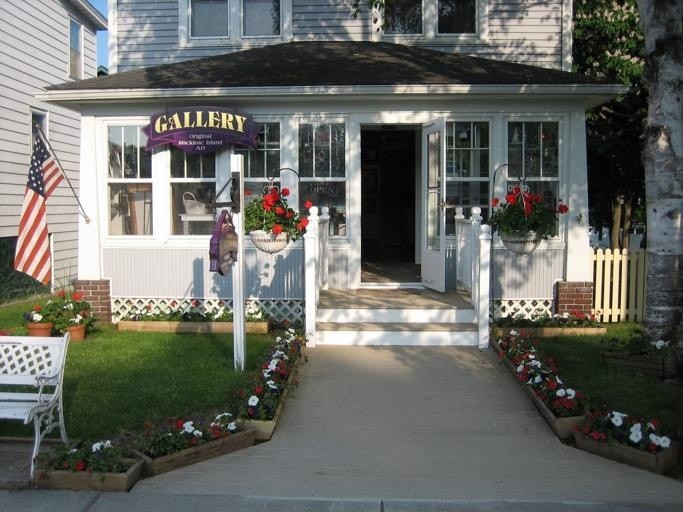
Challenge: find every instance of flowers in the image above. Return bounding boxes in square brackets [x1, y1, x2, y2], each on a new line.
[241, 185, 312, 237]
[22, 273, 96, 328]
[491, 185, 569, 239]
[493, 329, 668, 452]
[29, 327, 304, 471]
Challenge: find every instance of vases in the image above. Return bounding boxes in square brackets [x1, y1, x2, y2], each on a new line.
[44, 458, 141, 492]
[247, 229, 290, 254]
[25, 320, 85, 342]
[572, 428, 677, 474]
[498, 224, 543, 253]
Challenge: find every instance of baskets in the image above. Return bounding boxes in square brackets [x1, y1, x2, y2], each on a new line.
[497, 229, 542, 254]
[250, 232, 291, 254]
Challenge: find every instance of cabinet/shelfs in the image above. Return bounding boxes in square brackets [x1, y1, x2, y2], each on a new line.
[362, 162, 379, 243]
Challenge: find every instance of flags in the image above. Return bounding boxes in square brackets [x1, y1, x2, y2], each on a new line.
[14, 124, 66, 286]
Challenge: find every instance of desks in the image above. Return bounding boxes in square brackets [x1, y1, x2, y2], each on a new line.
[177, 213, 219, 234]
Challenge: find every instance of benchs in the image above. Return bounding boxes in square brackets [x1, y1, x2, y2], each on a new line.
[1, 336, 72, 476]
